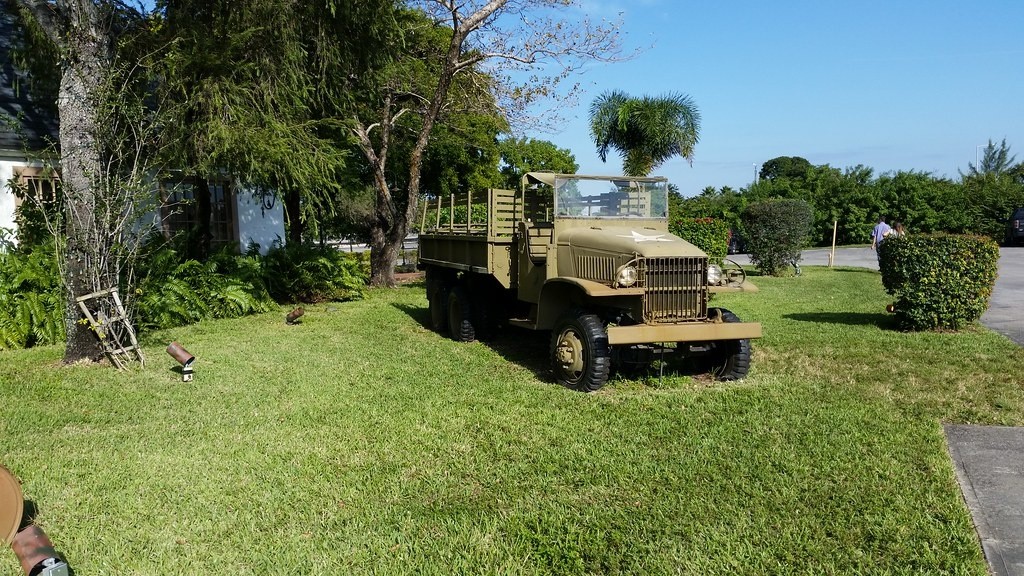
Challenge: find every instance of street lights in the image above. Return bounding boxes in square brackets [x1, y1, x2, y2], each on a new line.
[752, 161, 758, 184]
[976, 144, 996, 173]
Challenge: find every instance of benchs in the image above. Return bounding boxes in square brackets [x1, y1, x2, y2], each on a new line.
[528, 227, 553, 261]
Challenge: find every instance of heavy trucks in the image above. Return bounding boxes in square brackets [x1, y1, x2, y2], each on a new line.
[417, 170, 763, 391]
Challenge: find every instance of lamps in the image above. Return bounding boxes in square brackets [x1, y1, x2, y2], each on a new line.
[286, 307, 303, 325]
[10, 525, 69, 576]
[166, 340, 196, 382]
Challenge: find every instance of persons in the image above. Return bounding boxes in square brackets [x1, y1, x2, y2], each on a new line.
[870, 215, 894, 271]
[883, 222, 906, 238]
